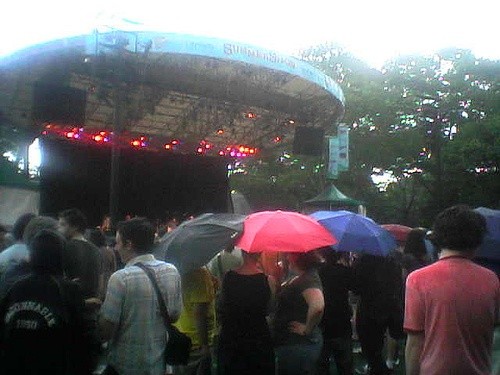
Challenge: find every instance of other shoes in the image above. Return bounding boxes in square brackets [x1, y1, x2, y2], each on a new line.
[353, 346, 363, 355]
[386, 358, 402, 371]
[355, 363, 372, 375]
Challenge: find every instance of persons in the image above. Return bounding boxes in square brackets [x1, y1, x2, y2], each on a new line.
[0, 210, 500, 375]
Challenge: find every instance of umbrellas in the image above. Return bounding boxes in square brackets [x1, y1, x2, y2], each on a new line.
[472, 207, 500, 261]
[234, 210, 336, 253]
[152, 213, 243, 271]
[375, 224, 413, 241]
[310, 210, 396, 256]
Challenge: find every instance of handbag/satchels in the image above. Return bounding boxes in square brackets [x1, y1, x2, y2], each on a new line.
[162, 326, 193, 366]
